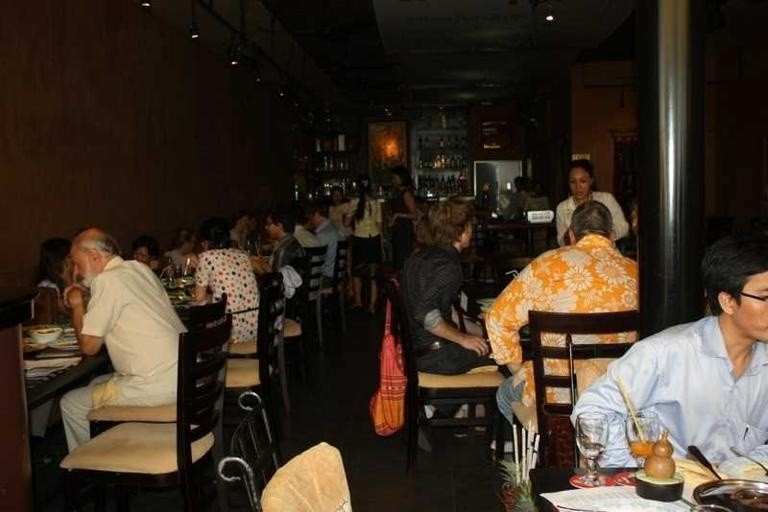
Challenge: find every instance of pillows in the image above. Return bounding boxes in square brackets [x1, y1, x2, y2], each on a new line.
[259, 442, 352, 512]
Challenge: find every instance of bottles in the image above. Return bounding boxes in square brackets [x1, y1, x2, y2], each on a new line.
[477, 298, 495, 304]
[183, 258, 192, 280]
[292, 111, 472, 200]
[167, 257, 176, 288]
[483, 181, 490, 209]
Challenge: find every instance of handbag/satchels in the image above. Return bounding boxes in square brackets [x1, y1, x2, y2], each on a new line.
[369, 335, 409, 437]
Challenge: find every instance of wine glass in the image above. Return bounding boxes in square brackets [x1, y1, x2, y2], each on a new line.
[626, 411, 660, 469]
[575, 410, 609, 486]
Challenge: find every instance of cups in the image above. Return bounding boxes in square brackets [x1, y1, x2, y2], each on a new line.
[691, 505, 732, 512]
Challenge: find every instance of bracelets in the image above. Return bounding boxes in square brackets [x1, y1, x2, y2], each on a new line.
[63, 283, 87, 308]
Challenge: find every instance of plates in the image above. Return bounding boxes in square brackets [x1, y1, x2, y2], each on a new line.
[64, 328, 76, 337]
[694, 479, 768, 512]
[718, 456, 768, 480]
[23, 337, 48, 353]
[49, 341, 80, 351]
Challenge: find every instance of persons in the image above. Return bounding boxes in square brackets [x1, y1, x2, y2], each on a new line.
[131, 166, 430, 342]
[29, 237, 81, 438]
[483, 200, 640, 465]
[569, 233, 768, 483]
[60, 228, 202, 452]
[400, 197, 515, 462]
[556, 160, 629, 246]
[496, 175, 549, 255]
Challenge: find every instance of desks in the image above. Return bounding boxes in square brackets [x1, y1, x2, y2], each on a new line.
[488, 220, 533, 256]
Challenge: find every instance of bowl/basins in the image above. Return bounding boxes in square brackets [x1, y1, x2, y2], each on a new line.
[30, 328, 62, 342]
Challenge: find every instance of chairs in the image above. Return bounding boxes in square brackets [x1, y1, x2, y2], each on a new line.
[320, 239, 349, 334]
[303, 244, 326, 350]
[228, 270, 294, 415]
[56, 312, 236, 512]
[458, 222, 489, 283]
[217, 392, 278, 511]
[527, 305, 642, 470]
[87, 293, 229, 437]
[383, 277, 506, 479]
